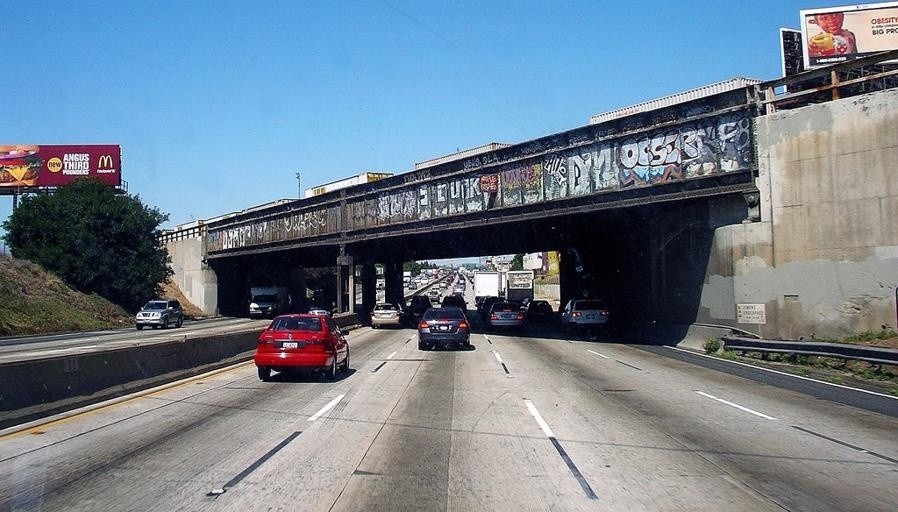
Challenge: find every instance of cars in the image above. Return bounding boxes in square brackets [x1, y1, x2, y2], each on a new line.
[475, 295, 611, 334]
[253, 313, 350, 380]
[368, 265, 475, 351]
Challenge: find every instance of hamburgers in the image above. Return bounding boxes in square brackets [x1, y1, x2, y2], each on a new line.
[0, 145, 43, 187]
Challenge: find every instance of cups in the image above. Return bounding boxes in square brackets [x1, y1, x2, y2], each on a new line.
[811, 33, 835, 52]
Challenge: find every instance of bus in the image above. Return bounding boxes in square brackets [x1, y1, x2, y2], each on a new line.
[505, 269, 535, 304]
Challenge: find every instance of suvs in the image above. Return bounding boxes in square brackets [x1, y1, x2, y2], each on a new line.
[134, 298, 185, 330]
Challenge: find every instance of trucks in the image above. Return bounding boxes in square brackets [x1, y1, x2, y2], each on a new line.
[246, 285, 288, 320]
[473, 271, 499, 298]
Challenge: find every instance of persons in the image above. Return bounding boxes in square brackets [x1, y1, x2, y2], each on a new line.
[807, 12, 859, 58]
